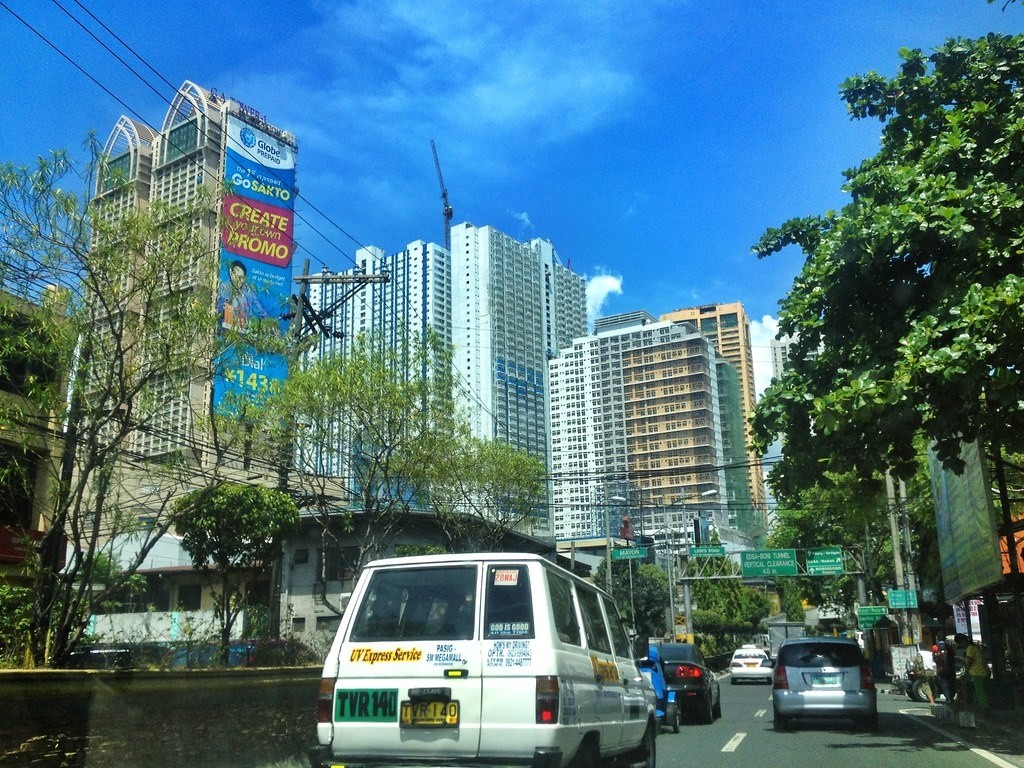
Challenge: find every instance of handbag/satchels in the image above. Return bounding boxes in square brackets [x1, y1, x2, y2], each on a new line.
[937, 650, 951, 677]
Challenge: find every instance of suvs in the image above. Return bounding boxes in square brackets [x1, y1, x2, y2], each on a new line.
[307, 552, 662, 768]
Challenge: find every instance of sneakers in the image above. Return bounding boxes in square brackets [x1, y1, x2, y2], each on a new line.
[947, 699, 954, 704]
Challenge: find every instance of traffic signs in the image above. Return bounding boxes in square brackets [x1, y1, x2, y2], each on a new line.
[741, 550, 798, 576]
[859, 606, 889, 629]
[805, 546, 844, 576]
[889, 590, 919, 608]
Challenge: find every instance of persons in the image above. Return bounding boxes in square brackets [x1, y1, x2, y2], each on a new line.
[913, 642, 937, 706]
[933, 638, 956, 704]
[954, 633, 995, 732]
[217, 260, 249, 340]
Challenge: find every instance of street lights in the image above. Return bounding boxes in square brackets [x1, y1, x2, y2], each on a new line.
[609, 490, 719, 643]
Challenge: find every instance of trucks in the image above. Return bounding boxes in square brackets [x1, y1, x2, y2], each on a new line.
[766, 621, 806, 661]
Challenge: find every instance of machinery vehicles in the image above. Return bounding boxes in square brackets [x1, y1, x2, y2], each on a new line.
[892, 637, 995, 702]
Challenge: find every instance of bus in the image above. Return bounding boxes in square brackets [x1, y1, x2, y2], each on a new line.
[818, 617, 859, 639]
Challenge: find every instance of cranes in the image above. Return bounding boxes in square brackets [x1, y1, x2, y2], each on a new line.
[429, 138, 455, 250]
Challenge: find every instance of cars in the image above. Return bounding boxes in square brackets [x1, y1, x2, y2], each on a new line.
[653, 642, 720, 725]
[729, 644, 773, 683]
[856, 632, 865, 649]
[771, 637, 879, 731]
